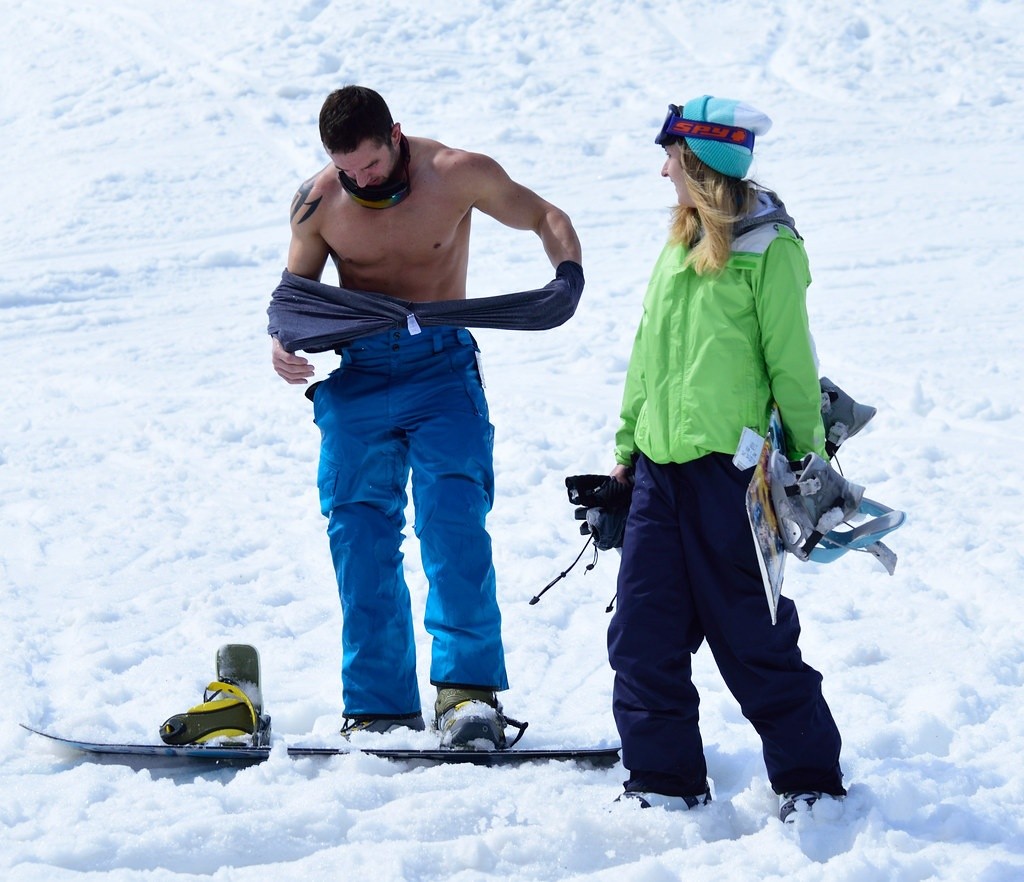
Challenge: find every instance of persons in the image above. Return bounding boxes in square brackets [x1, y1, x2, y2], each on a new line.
[271, 84, 587, 748]
[603, 96, 850, 824]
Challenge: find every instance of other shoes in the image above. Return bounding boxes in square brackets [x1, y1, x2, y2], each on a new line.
[616, 786, 714, 814]
[778, 792, 844, 823]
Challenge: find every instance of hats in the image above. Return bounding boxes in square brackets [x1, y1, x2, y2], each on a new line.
[682, 95, 771, 179]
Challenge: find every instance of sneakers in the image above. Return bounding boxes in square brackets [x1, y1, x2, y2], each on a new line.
[341, 715, 425, 745]
[436, 684, 507, 749]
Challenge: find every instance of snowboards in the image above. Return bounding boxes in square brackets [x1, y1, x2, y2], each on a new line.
[739, 376, 877, 629]
[10, 721, 624, 765]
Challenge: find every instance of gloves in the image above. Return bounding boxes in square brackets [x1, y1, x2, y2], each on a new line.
[565, 474, 634, 549]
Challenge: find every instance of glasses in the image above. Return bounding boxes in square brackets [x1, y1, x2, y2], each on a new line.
[337, 132, 411, 210]
[654, 103, 755, 152]
